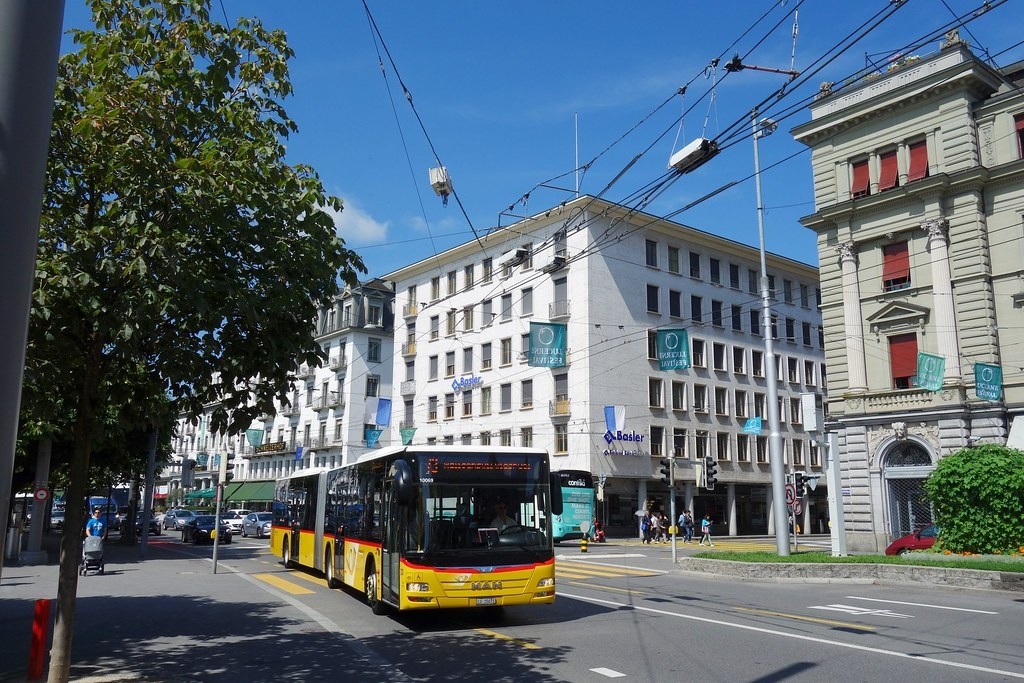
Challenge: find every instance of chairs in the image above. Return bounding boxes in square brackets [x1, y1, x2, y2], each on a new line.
[429, 514, 471, 546]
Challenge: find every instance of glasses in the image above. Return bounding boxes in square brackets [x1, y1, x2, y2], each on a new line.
[499, 507, 506, 509]
[95, 512, 100, 514]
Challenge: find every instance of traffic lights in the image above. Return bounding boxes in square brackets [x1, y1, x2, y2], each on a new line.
[705, 456, 718, 490]
[226, 453, 236, 481]
[795, 473, 805, 497]
[660, 459, 670, 485]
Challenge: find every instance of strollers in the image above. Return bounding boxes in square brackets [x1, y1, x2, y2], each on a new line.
[79, 536, 104, 577]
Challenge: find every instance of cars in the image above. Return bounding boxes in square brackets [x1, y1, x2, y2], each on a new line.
[219, 513, 243, 533]
[120, 510, 162, 536]
[227, 509, 252, 519]
[11, 493, 211, 531]
[884, 521, 939, 556]
[240, 512, 272, 539]
[181, 515, 232, 545]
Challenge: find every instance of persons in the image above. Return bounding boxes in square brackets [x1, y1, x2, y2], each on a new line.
[86, 508, 107, 540]
[641, 509, 693, 545]
[489, 502, 518, 535]
[698, 514, 714, 547]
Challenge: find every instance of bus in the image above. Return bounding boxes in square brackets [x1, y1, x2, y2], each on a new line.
[520, 469, 595, 543]
[269, 445, 564, 614]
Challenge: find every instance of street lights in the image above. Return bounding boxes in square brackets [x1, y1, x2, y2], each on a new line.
[751, 112, 791, 556]
